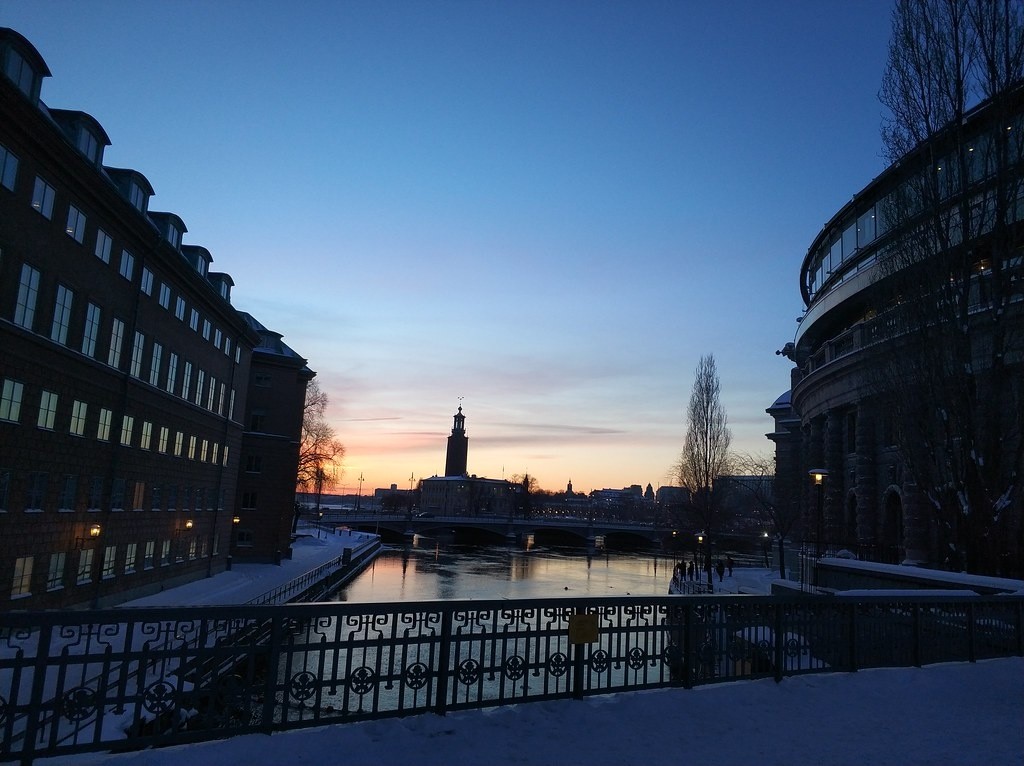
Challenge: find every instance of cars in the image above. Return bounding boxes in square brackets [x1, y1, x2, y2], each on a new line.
[416, 512, 435, 517]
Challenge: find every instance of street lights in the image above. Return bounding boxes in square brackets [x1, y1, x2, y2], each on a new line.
[809, 468, 831, 558]
[672, 529, 677, 575]
[695, 533, 706, 584]
[763, 531, 770, 568]
